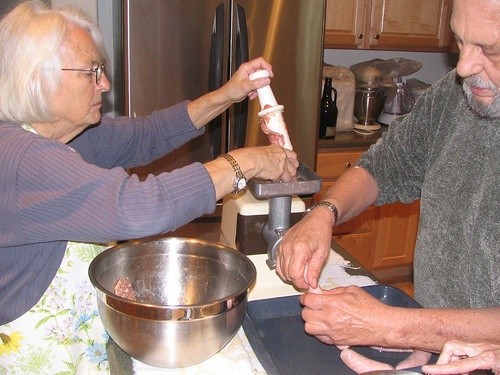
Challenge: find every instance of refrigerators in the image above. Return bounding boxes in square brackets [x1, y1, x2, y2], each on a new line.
[95, 0, 326, 247]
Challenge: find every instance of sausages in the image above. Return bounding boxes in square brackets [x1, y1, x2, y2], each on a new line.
[308, 284, 431, 373]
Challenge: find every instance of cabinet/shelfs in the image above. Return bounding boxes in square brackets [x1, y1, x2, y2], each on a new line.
[315, 152, 421, 284]
[323, 0, 448, 54]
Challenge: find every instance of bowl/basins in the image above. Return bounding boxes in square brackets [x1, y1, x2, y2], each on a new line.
[88, 235, 257, 367]
[354, 87, 387, 126]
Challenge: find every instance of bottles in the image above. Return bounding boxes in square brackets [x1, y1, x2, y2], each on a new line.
[321, 77, 338, 139]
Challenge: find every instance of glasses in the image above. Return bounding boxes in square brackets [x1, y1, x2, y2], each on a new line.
[45, 58, 106, 85]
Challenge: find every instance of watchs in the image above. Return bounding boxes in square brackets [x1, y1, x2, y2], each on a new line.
[217, 153, 247, 194]
[302, 201, 338, 224]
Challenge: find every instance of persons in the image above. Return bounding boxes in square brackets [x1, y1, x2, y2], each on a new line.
[275, 1, 499, 375]
[0, 0, 302, 325]
